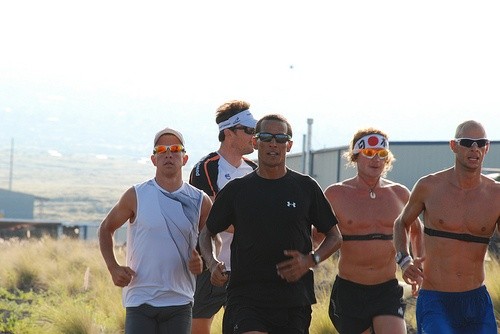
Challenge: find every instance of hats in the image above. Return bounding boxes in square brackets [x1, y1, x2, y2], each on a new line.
[218, 110, 258, 132]
[154, 128, 183, 146]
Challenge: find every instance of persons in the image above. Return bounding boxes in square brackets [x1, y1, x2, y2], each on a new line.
[189, 100, 260, 334]
[393, 120, 500, 334]
[311, 128, 425, 334]
[98, 126, 222, 334]
[198, 114, 343, 333]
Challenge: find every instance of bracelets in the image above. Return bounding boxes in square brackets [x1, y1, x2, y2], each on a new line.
[400, 255, 413, 269]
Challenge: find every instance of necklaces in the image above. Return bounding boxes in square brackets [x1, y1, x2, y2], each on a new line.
[357, 173, 380, 200]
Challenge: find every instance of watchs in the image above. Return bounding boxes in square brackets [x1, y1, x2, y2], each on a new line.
[394, 251, 411, 265]
[309, 250, 320, 268]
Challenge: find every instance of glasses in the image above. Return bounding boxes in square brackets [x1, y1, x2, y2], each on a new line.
[454, 137, 489, 148]
[255, 133, 292, 144]
[355, 149, 389, 159]
[155, 144, 184, 153]
[230, 126, 253, 135]
